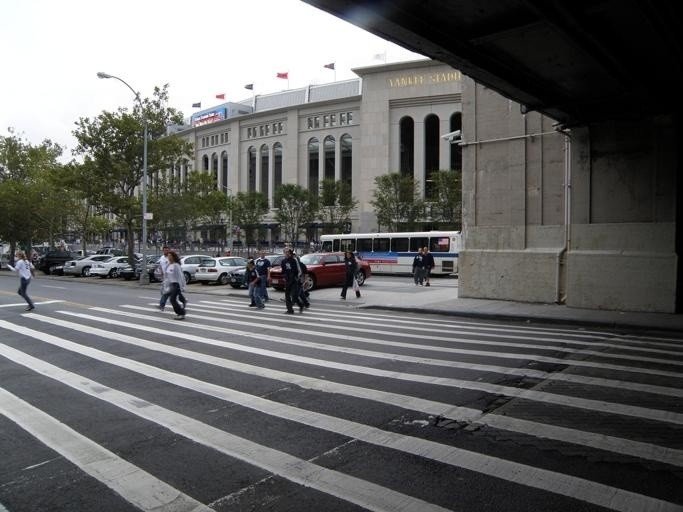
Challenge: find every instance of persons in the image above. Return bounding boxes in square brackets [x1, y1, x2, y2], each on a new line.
[215, 251, 220, 257]
[5, 251, 35, 311]
[411, 248, 427, 287]
[339, 252, 361, 300]
[155, 246, 186, 312]
[226, 250, 231, 256]
[165, 251, 186, 320]
[421, 246, 435, 287]
[244, 243, 310, 316]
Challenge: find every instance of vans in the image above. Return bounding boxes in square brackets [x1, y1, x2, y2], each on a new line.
[95, 247, 123, 256]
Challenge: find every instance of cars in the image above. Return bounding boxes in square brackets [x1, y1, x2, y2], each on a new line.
[223, 255, 284, 290]
[89, 256, 134, 279]
[194, 256, 249, 285]
[134, 255, 161, 280]
[119, 253, 153, 280]
[62, 254, 113, 276]
[267, 251, 370, 291]
[153, 254, 228, 284]
[0, 244, 96, 279]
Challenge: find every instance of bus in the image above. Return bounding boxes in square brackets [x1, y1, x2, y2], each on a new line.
[317, 231, 460, 278]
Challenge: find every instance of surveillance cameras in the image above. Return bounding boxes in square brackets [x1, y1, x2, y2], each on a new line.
[442, 129, 461, 142]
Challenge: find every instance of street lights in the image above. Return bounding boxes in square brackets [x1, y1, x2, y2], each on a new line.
[218, 181, 233, 255]
[97, 70, 150, 287]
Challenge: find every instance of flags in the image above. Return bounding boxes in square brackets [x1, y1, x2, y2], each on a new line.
[191, 103, 200, 108]
[323, 63, 334, 69]
[276, 72, 287, 80]
[215, 94, 224, 99]
[243, 84, 253, 90]
